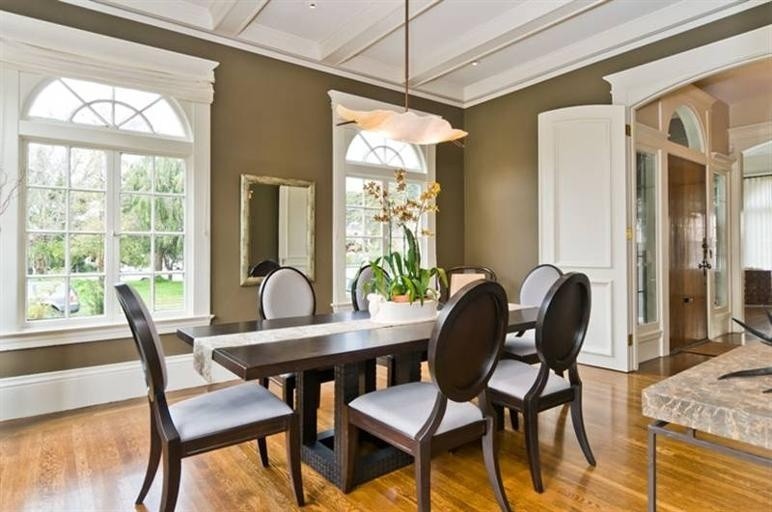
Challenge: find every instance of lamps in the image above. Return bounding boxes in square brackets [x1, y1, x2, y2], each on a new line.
[336, 0, 471, 145]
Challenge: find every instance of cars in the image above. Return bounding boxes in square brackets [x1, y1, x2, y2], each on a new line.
[26, 281, 78, 314]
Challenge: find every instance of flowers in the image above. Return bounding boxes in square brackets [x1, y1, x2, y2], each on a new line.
[359, 164, 449, 308]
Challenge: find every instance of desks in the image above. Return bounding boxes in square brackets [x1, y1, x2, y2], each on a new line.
[639, 340, 772, 512]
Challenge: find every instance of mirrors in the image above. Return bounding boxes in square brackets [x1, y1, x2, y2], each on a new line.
[239, 174, 317, 287]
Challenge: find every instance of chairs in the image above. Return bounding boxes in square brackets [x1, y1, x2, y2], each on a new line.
[249, 259, 280, 278]
